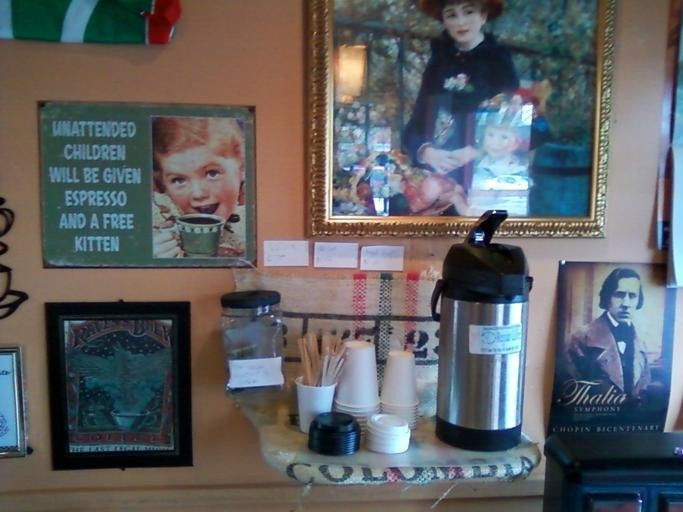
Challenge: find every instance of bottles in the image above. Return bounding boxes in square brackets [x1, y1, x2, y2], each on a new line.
[428, 204, 534, 453]
[219, 288, 287, 399]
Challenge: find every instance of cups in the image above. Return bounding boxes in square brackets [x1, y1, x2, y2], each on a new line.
[333, 340, 381, 440]
[295, 374, 340, 435]
[380, 349, 420, 431]
[158, 214, 229, 258]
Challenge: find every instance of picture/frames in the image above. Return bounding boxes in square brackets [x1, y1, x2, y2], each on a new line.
[301, 2, 617, 240]
[44, 299, 193, 472]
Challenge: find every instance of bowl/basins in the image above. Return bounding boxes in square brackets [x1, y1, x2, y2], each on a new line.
[363, 413, 412, 455]
[306, 412, 361, 457]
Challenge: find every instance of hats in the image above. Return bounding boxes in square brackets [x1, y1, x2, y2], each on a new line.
[416, 0, 502, 21]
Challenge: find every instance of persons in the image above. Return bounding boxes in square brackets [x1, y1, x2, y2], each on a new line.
[472, 123, 529, 191]
[401, 0, 519, 192]
[561, 268, 651, 408]
[152, 116, 246, 258]
